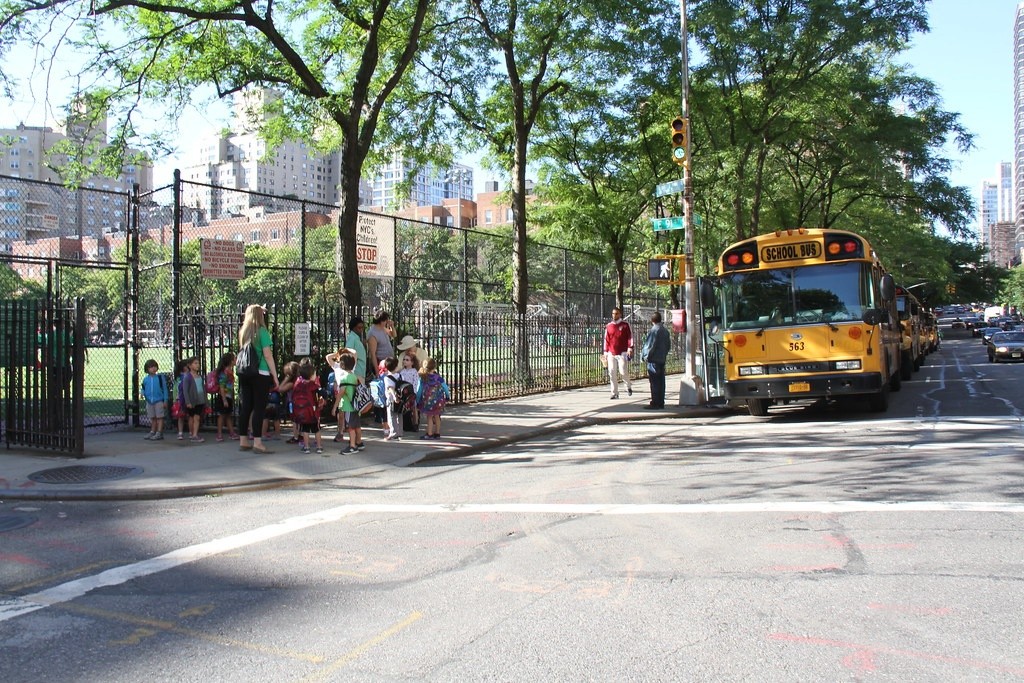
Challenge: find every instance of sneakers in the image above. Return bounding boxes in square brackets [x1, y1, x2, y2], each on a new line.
[144, 425, 440, 454]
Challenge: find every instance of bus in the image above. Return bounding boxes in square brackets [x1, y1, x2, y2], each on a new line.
[700, 227, 899, 415]
[895, 282, 944, 380]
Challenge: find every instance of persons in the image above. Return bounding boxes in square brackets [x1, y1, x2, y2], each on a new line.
[30, 320, 89, 387]
[278, 357, 325, 454]
[549, 331, 562, 354]
[326, 310, 451, 455]
[140, 351, 237, 442]
[934, 326, 944, 353]
[603, 307, 634, 399]
[640, 312, 671, 410]
[236, 304, 279, 454]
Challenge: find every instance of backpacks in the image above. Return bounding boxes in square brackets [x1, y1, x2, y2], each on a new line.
[388, 374, 416, 414]
[291, 379, 320, 425]
[236, 341, 263, 379]
[326, 372, 335, 396]
[205, 367, 233, 393]
[419, 373, 444, 415]
[339, 378, 372, 417]
[178, 373, 193, 412]
[264, 385, 280, 420]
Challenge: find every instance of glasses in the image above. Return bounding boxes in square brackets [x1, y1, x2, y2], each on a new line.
[612, 313, 620, 315]
[403, 359, 412, 361]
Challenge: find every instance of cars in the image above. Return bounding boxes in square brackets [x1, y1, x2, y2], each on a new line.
[986, 330, 1024, 363]
[947, 304, 1024, 338]
[90, 328, 230, 347]
[952, 318, 965, 329]
[981, 328, 1004, 345]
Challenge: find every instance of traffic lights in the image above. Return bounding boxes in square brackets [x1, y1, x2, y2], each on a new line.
[671, 117, 689, 163]
[646, 258, 672, 282]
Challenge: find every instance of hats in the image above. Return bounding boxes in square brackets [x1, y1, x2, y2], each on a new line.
[397, 336, 419, 350]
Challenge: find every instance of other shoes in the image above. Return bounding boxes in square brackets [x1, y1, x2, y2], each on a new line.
[644, 401, 664, 410]
[611, 395, 619, 399]
[628, 389, 632, 396]
